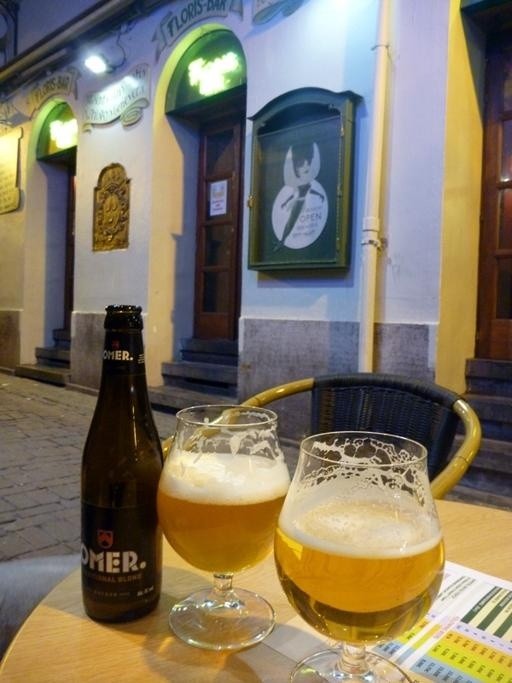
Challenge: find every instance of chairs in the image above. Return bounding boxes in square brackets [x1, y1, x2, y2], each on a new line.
[156, 373, 482, 501]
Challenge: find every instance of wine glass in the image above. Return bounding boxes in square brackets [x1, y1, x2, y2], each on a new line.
[274, 430, 446, 683]
[152, 404, 289, 653]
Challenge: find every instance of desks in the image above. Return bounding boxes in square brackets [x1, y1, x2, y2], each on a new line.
[0, 500, 512, 683]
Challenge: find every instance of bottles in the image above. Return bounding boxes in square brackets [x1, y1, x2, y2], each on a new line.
[80, 304, 165, 623]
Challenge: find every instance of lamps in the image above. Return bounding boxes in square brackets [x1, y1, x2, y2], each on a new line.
[85, 26, 126, 75]
